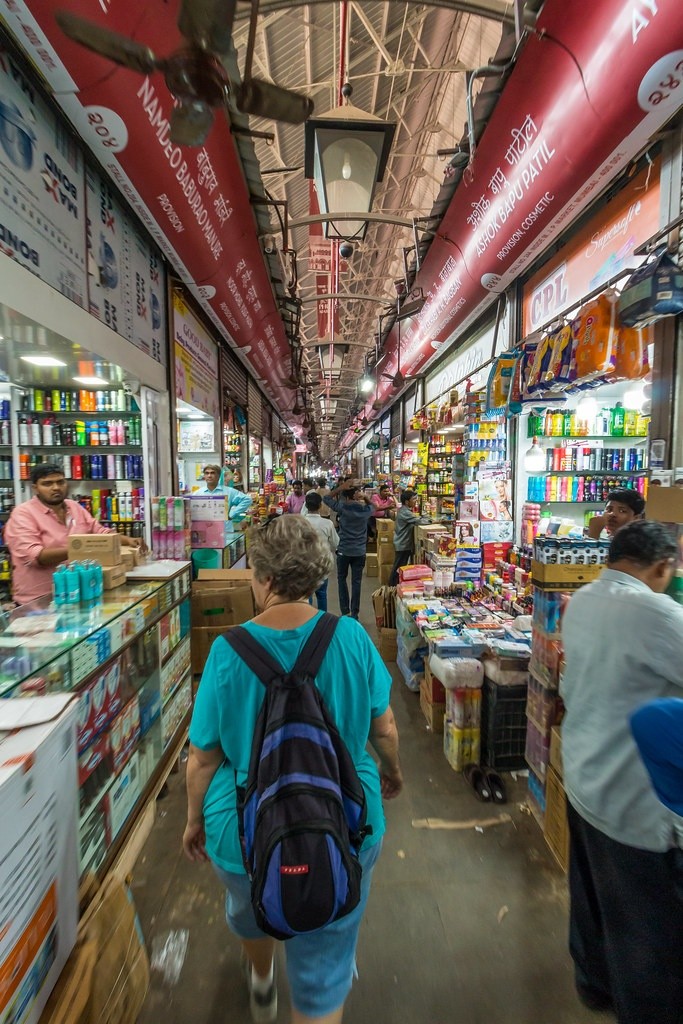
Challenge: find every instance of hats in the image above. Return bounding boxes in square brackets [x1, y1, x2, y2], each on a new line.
[30, 462, 64, 484]
[400, 491, 418, 502]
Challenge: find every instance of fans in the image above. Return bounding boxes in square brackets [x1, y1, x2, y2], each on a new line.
[55, 0, 316, 149]
[357, 292, 428, 427]
[278, 248, 327, 438]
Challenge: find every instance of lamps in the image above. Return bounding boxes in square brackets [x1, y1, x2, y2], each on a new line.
[359, 346, 382, 392]
[282, 295, 303, 316]
[439, 144, 469, 180]
[305, 83, 397, 256]
[288, 335, 302, 348]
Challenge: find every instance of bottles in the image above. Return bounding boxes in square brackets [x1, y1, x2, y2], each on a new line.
[1, 420, 10, 445]
[223, 434, 241, 452]
[20, 388, 140, 411]
[584, 509, 605, 526]
[18, 414, 141, 446]
[428, 456, 453, 467]
[0, 488, 15, 513]
[528, 475, 648, 502]
[429, 482, 454, 494]
[527, 402, 649, 438]
[486, 544, 533, 597]
[21, 453, 144, 479]
[429, 434, 466, 454]
[224, 453, 240, 466]
[51, 559, 103, 604]
[524, 436, 546, 470]
[428, 470, 452, 482]
[0, 455, 12, 480]
[232, 468, 241, 484]
[546, 448, 643, 471]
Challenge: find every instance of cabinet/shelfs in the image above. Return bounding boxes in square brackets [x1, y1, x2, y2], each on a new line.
[0, 559, 195, 886]
[426, 453, 454, 497]
[526, 433, 647, 504]
[225, 430, 263, 492]
[190, 531, 247, 576]
[0, 384, 159, 581]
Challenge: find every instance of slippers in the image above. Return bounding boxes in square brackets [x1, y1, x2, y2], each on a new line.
[479, 762, 507, 804]
[463, 762, 492, 803]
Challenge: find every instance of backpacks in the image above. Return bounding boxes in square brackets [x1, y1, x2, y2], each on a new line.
[221, 611, 374, 942]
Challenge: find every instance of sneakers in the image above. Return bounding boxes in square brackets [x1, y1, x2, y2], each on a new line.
[238, 943, 278, 1024]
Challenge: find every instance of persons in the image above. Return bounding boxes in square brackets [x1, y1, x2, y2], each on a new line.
[323, 479, 378, 620]
[388, 490, 435, 585]
[459, 522, 473, 545]
[367, 484, 395, 543]
[4, 463, 144, 606]
[589, 488, 645, 540]
[182, 515, 403, 1024]
[558, 520, 683, 1024]
[301, 492, 340, 612]
[499, 501, 512, 520]
[494, 479, 508, 501]
[187, 464, 253, 520]
[285, 476, 352, 529]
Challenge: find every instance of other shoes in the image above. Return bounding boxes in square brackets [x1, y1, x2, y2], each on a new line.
[368, 536, 374, 543]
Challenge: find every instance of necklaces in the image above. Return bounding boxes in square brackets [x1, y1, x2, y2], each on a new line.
[264, 600, 309, 609]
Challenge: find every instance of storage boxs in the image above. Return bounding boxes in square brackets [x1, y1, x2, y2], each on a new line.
[524, 586, 571, 874]
[365, 479, 531, 770]
[646, 484, 683, 524]
[0, 582, 198, 1024]
[530, 558, 605, 592]
[176, 419, 228, 548]
[67, 533, 148, 588]
[190, 569, 262, 693]
